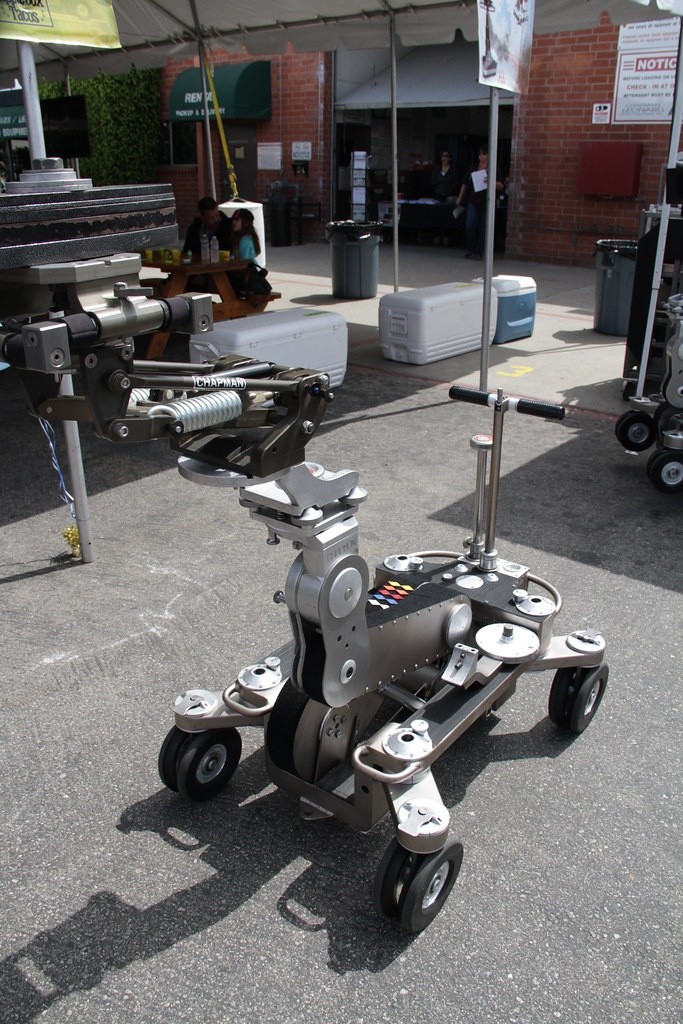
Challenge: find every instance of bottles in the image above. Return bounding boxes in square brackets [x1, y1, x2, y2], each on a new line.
[187, 248, 192, 259]
[210, 236, 219, 264]
[168, 253, 173, 261]
[201, 233, 210, 261]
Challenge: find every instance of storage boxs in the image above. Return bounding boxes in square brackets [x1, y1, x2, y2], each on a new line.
[477, 274, 535, 344]
[189, 307, 348, 392]
[380, 280, 497, 365]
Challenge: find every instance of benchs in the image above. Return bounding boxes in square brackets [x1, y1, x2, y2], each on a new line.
[233, 291, 281, 304]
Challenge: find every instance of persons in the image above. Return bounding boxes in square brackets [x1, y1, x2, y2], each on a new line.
[181, 197, 261, 304]
[432, 147, 503, 258]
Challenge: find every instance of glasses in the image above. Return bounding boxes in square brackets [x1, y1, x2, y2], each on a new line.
[440, 155, 448, 157]
[477, 151, 487, 155]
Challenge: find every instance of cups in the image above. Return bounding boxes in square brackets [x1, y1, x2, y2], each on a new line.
[220, 250, 230, 261]
[144, 247, 179, 260]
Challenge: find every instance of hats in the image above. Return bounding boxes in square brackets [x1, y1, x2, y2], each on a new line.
[227, 209, 254, 220]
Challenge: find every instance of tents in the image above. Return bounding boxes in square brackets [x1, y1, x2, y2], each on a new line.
[0, 1, 682, 564]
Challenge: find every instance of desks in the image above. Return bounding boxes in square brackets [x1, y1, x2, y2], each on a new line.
[139, 257, 253, 361]
[380, 200, 456, 248]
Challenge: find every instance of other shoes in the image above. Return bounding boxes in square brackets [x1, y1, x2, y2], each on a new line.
[474, 252, 481, 261]
[464, 252, 472, 258]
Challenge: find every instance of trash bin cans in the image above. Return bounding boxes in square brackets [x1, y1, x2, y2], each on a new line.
[594, 239, 635, 337]
[326, 220, 378, 298]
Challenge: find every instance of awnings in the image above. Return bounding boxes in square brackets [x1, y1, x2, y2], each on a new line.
[167, 60, 272, 122]
